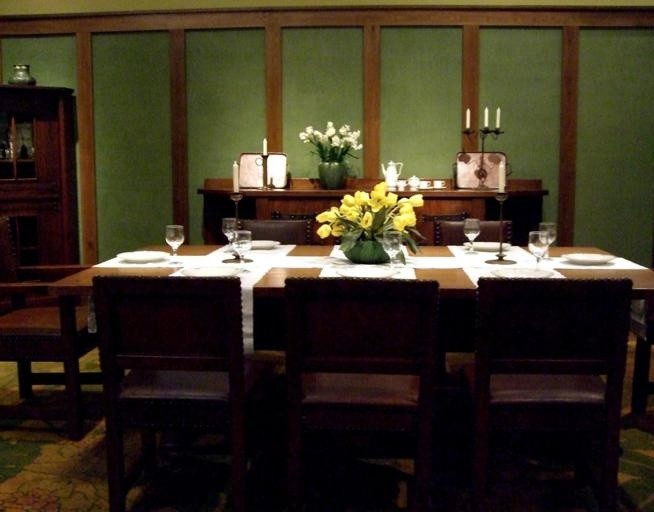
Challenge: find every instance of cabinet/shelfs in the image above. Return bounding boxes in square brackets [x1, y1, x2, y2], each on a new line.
[1, 82, 75, 307]
[196, 177, 549, 247]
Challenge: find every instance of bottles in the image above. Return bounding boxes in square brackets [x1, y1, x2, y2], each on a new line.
[408, 176, 419, 186]
[0, 139, 29, 158]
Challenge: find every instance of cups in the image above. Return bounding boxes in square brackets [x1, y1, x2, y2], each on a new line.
[398, 180, 408, 190]
[434, 181, 445, 188]
[418, 181, 431, 188]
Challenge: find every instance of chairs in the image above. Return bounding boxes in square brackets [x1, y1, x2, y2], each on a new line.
[0, 213, 99, 443]
[236, 218, 312, 247]
[432, 215, 512, 244]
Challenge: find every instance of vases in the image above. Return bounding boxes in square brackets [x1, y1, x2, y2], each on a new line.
[318, 161, 343, 188]
[340, 238, 397, 264]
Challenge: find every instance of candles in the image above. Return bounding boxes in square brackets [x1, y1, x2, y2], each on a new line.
[497, 160, 505, 193]
[261, 137, 268, 156]
[465, 108, 471, 128]
[231, 160, 240, 193]
[483, 107, 501, 129]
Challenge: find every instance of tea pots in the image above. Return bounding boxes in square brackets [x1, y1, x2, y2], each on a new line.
[380, 161, 404, 191]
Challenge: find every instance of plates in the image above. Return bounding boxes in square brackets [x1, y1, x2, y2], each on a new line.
[252, 239, 281, 251]
[464, 242, 511, 252]
[432, 187, 447, 190]
[417, 187, 433, 189]
[118, 250, 169, 262]
[562, 253, 615, 265]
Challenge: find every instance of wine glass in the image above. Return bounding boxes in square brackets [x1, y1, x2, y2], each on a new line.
[222, 218, 237, 252]
[164, 225, 186, 266]
[463, 219, 481, 254]
[540, 223, 557, 260]
[233, 231, 253, 273]
[528, 231, 549, 272]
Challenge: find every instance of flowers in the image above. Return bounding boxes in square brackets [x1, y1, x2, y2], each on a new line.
[313, 181, 428, 255]
[297, 120, 363, 164]
